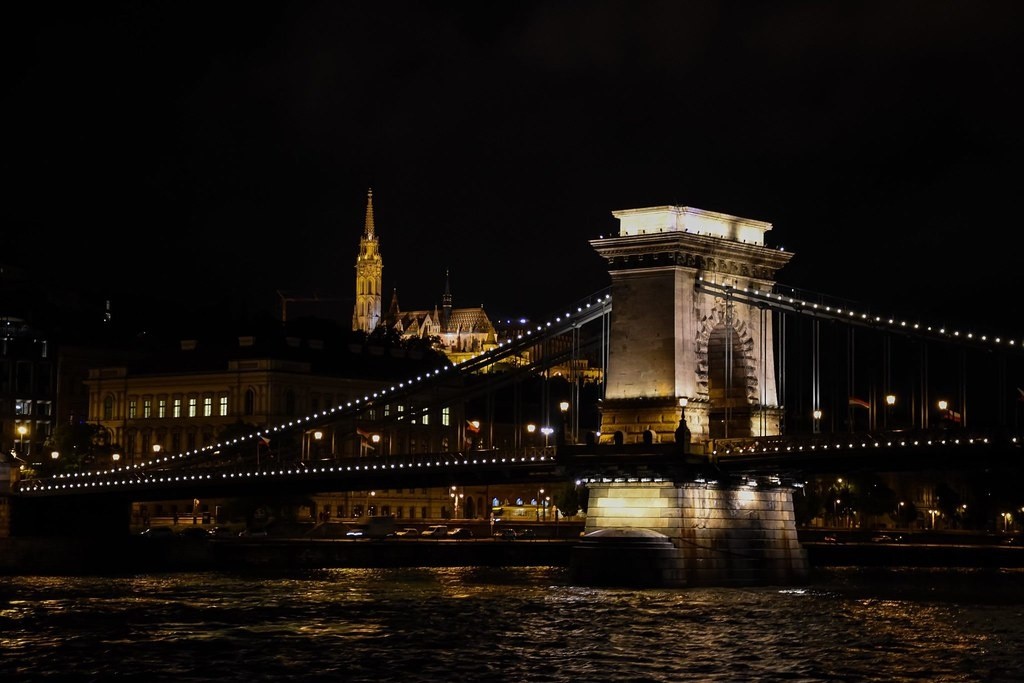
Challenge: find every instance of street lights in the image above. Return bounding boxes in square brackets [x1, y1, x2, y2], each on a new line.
[886, 393, 895, 434]
[537, 488, 544, 521]
[371, 432, 380, 455]
[1002, 513, 1010, 532]
[674, 395, 691, 443]
[937, 400, 947, 432]
[543, 497, 550, 521]
[468, 421, 478, 451]
[19, 427, 25, 451]
[153, 444, 160, 460]
[112, 452, 119, 471]
[556, 400, 571, 445]
[897, 502, 904, 517]
[833, 500, 840, 527]
[50, 451, 59, 475]
[929, 510, 937, 528]
[312, 429, 322, 460]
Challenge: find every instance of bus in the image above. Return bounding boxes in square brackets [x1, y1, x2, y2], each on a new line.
[492, 505, 552, 522]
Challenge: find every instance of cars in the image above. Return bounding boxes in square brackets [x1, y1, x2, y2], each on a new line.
[207, 527, 235, 538]
[872, 535, 889, 542]
[493, 529, 517, 540]
[883, 425, 909, 433]
[516, 529, 537, 539]
[1001, 538, 1018, 546]
[452, 529, 479, 539]
[176, 527, 209, 539]
[346, 529, 366, 537]
[239, 528, 268, 537]
[394, 528, 418, 537]
[140, 527, 172, 537]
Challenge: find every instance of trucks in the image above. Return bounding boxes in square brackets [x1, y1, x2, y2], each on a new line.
[421, 525, 447, 538]
